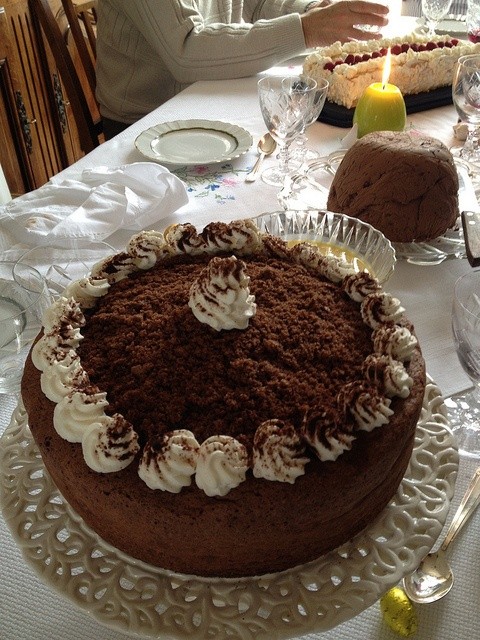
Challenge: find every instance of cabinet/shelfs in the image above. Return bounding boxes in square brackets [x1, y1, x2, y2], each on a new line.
[0, 0, 98, 203]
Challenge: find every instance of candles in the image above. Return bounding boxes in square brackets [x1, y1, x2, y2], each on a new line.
[352, 47, 406, 139]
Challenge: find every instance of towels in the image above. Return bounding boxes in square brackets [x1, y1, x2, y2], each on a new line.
[2, 163, 188, 250]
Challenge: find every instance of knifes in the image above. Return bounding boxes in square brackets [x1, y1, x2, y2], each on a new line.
[451, 160, 480, 267]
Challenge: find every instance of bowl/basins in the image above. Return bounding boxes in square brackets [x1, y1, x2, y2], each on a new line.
[227, 206, 396, 306]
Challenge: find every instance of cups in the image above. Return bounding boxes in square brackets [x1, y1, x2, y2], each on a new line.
[10, 237, 135, 313]
[1, 258, 49, 402]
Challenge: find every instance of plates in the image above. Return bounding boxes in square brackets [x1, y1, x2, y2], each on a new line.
[131, 118, 254, 170]
[277, 146, 466, 264]
[0, 366, 460, 638]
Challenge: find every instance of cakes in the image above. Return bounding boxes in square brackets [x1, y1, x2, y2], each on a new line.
[306, 32, 480, 109]
[20, 219, 426, 577]
[329, 130, 460, 243]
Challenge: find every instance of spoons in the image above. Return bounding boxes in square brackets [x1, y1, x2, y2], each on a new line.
[246, 122, 280, 185]
[404, 455, 480, 605]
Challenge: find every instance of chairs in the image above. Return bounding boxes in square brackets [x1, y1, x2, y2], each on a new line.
[32, 1, 113, 150]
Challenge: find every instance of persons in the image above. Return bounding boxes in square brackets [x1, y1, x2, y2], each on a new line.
[94, 0, 390, 141]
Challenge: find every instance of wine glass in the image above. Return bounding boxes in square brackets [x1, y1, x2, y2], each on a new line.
[461, 1, 480, 49]
[255, 71, 330, 186]
[443, 268, 480, 457]
[447, 49, 480, 162]
[415, 0, 454, 40]
